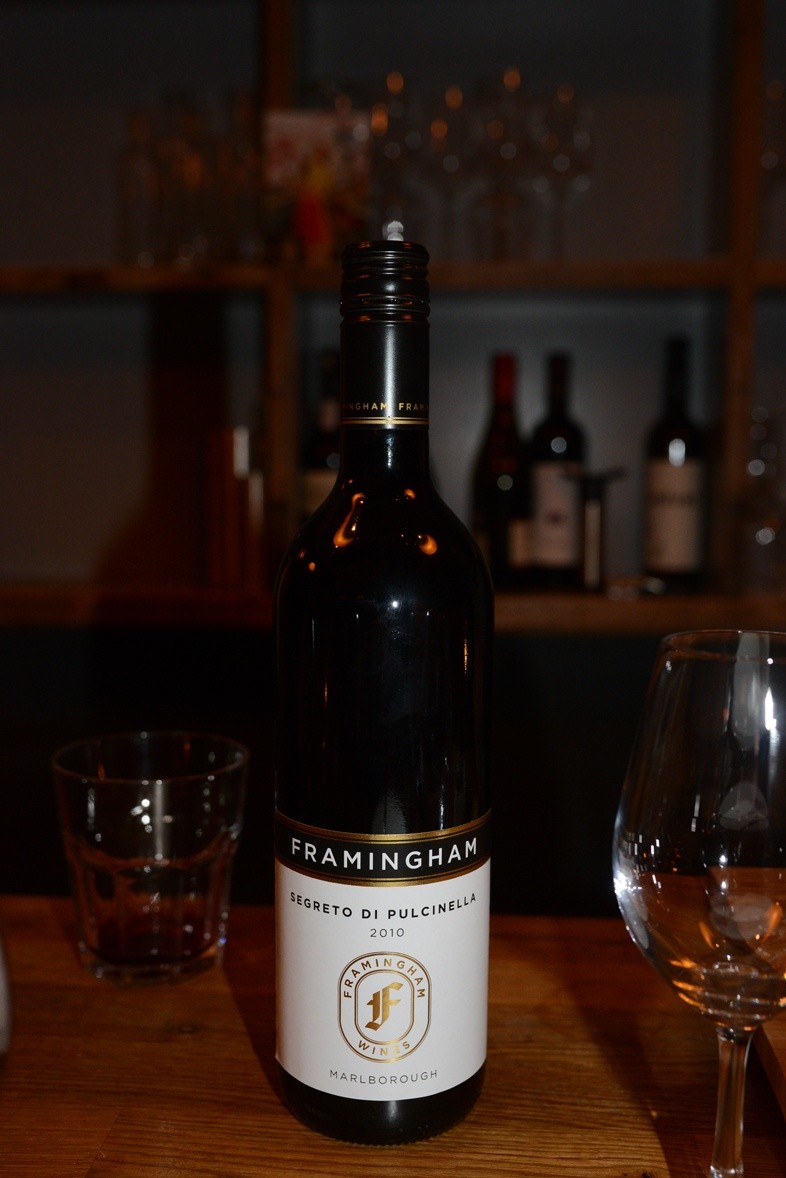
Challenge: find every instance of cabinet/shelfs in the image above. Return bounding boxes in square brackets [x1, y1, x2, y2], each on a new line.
[0, 0, 786, 638]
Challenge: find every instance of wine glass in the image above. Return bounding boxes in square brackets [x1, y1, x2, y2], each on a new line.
[612, 630, 786, 1178]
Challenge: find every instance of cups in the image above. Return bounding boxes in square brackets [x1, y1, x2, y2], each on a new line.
[51, 732, 249, 988]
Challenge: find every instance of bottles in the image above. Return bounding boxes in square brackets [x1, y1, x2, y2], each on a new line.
[513, 348, 605, 597]
[637, 335, 715, 597]
[273, 238, 493, 1148]
[465, 352, 532, 597]
